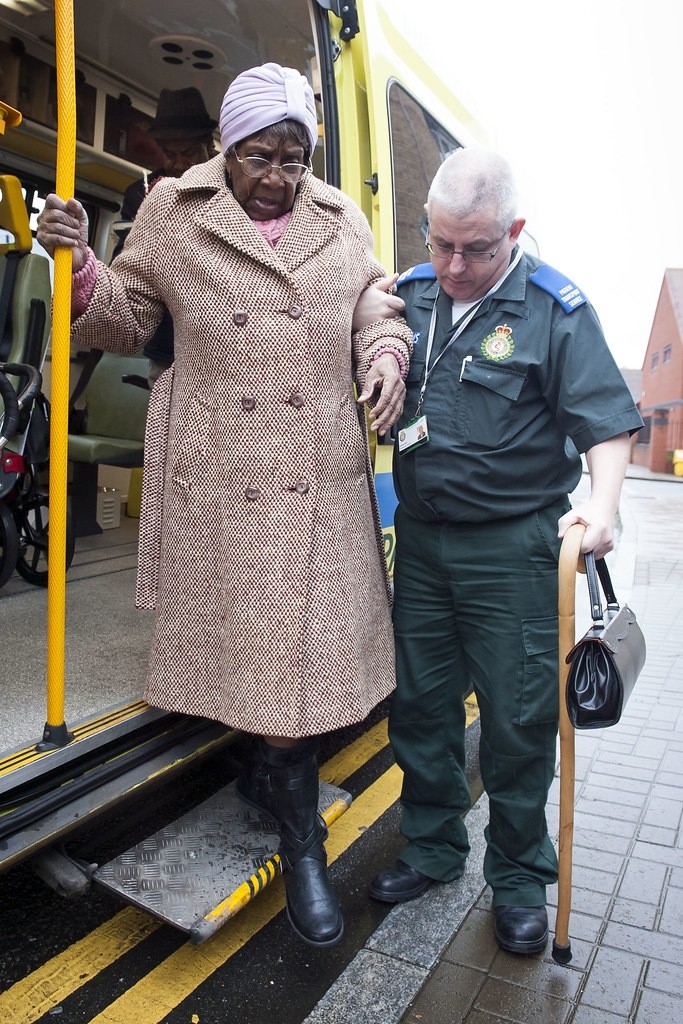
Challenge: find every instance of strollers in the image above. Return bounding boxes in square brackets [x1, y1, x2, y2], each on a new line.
[0, 358, 76, 591]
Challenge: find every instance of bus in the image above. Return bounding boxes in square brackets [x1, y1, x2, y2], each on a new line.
[0, 1, 565, 947]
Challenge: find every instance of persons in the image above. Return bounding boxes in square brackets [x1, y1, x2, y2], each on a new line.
[417, 426, 426, 440]
[37, 62, 416, 952]
[108, 85, 221, 391]
[350, 149, 647, 957]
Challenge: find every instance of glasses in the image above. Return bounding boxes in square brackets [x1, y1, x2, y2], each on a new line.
[232, 146, 313, 183]
[424, 224, 511, 263]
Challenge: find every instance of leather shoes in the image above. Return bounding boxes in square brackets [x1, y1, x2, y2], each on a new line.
[369, 858, 432, 902]
[493, 904, 549, 954]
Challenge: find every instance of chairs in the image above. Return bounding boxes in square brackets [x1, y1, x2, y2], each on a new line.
[0, 175, 52, 497]
[69, 350, 153, 537]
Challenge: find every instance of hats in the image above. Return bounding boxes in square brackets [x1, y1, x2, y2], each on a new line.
[220, 63, 318, 158]
[145, 87, 218, 139]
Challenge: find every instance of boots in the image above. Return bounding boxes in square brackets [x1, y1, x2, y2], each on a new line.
[263, 738, 344, 947]
[237, 731, 329, 843]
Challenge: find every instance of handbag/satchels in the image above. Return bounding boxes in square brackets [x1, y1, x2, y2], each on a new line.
[565, 550, 647, 729]
